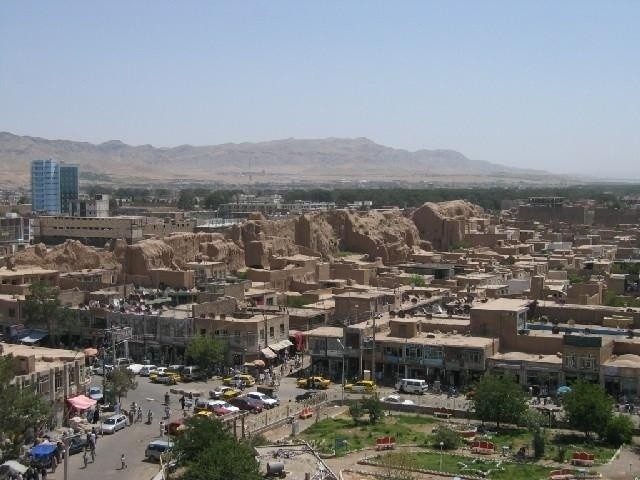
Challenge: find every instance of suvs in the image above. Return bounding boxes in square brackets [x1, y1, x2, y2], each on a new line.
[345, 380, 376, 394]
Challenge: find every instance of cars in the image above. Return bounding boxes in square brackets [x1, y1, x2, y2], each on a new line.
[298, 376, 331, 390]
[89, 386, 103, 403]
[375, 311, 384, 319]
[297, 391, 317, 404]
[165, 374, 281, 436]
[379, 395, 415, 407]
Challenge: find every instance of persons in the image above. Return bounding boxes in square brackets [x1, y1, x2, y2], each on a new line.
[120, 391, 200, 424]
[87, 409, 99, 425]
[120, 453, 127, 470]
[261, 352, 301, 387]
[6, 455, 57, 480]
[81, 426, 98, 468]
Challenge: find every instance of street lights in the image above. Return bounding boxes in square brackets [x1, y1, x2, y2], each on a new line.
[337, 338, 345, 405]
[354, 303, 359, 323]
[438, 441, 444, 471]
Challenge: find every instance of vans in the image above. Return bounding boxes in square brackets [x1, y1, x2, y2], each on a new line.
[399, 378, 429, 395]
[91, 358, 201, 384]
[145, 440, 173, 462]
[98, 414, 128, 433]
